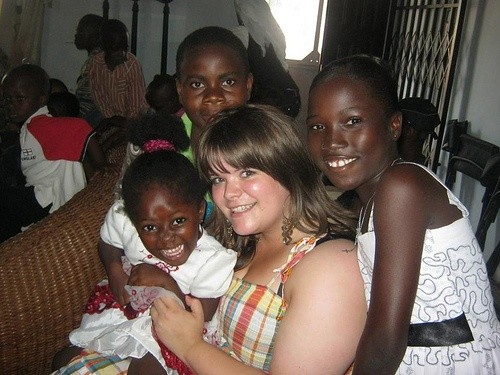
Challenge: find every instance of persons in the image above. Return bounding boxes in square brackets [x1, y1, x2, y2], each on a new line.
[0, 12, 500, 375]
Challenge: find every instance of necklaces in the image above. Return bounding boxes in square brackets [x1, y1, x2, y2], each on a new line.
[340, 157, 404, 253]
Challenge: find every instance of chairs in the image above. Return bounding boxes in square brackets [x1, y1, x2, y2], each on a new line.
[397, 97, 500, 320]
